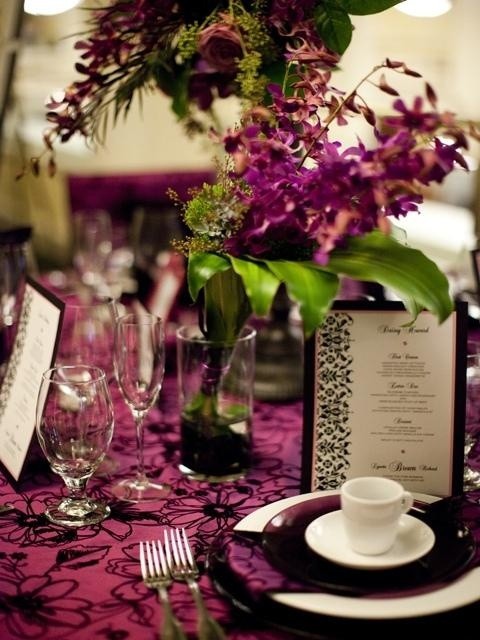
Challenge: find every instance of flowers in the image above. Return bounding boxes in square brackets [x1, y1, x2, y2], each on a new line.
[17, 0, 476, 470]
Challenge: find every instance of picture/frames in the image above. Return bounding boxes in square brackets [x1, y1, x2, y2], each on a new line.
[300, 296, 470, 507]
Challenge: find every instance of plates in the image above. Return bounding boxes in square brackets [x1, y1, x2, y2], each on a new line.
[233, 490, 480, 620]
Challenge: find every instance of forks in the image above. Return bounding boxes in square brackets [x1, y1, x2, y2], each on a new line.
[138, 528, 227, 640]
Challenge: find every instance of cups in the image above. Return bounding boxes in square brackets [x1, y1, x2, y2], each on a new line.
[340, 477, 414, 553]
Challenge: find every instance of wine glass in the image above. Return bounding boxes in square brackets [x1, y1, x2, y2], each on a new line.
[34, 293, 171, 529]
